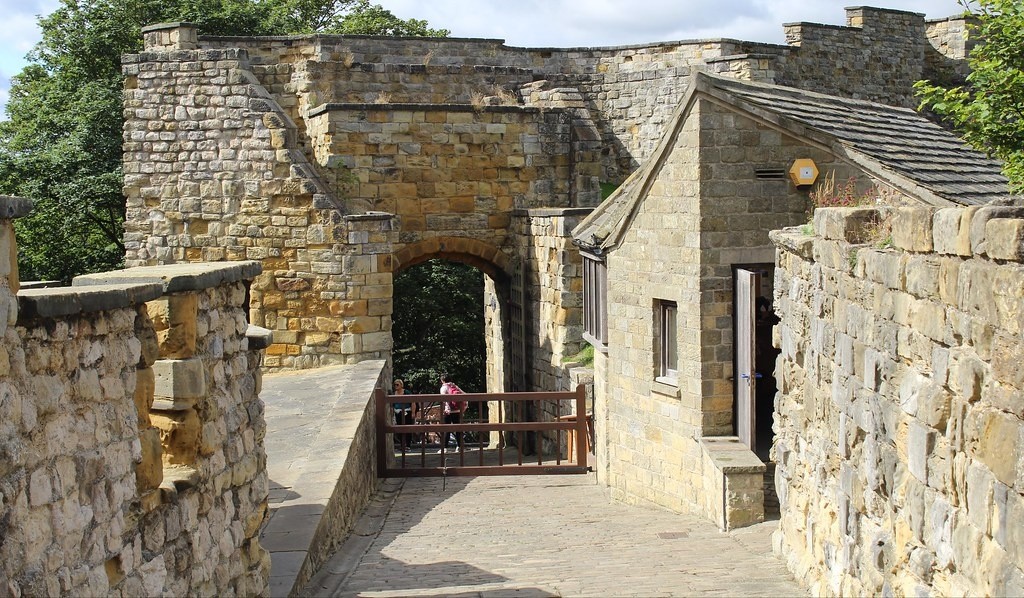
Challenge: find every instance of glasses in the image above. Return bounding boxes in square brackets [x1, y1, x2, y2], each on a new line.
[394, 384, 401, 386]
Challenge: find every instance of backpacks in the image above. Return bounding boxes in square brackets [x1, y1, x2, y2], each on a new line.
[443, 384, 466, 411]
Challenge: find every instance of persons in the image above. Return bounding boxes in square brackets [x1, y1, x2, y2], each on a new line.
[392, 379, 417, 453]
[437, 373, 467, 454]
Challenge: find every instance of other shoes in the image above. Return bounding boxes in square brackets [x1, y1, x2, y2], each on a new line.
[437, 448, 447, 453]
[454, 446, 460, 452]
